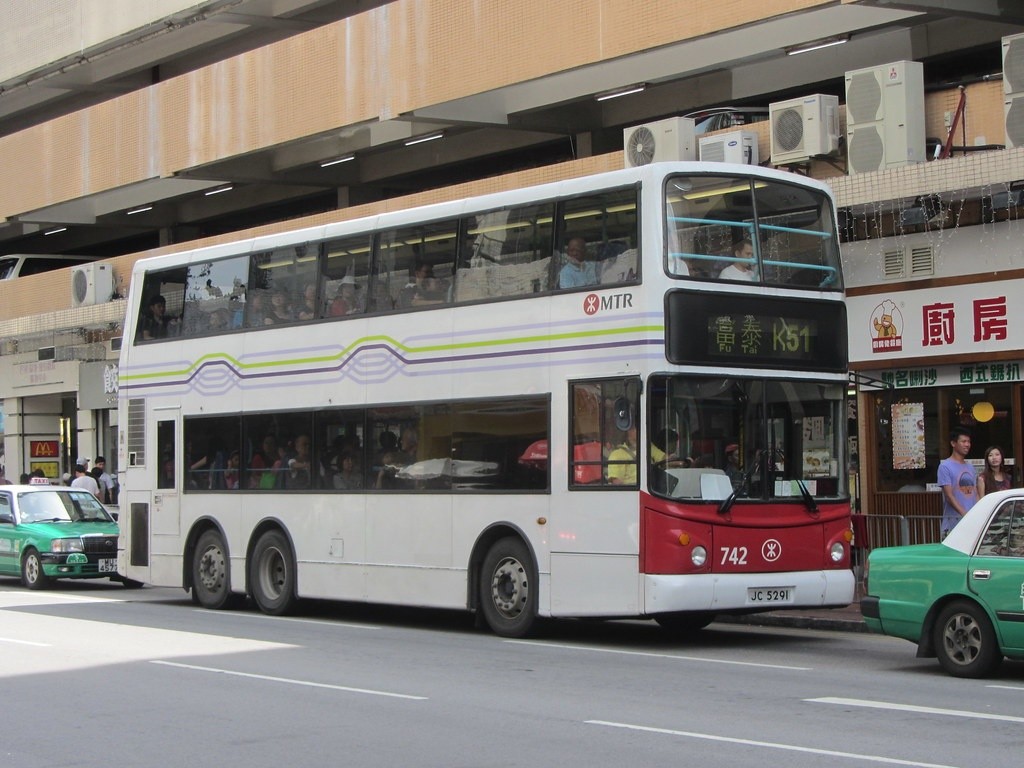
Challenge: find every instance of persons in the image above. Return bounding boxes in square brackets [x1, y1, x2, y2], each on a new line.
[559, 237, 596, 289]
[719, 240, 760, 281]
[403, 262, 443, 305]
[724, 444, 739, 473]
[95, 456, 115, 504]
[143, 283, 315, 340]
[937, 427, 978, 542]
[0, 468, 12, 485]
[91, 467, 108, 504]
[20, 469, 45, 484]
[330, 283, 362, 316]
[163, 427, 418, 489]
[977, 446, 1011, 542]
[657, 428, 679, 454]
[448, 261, 471, 303]
[67, 457, 100, 489]
[608, 422, 695, 484]
[71, 465, 102, 501]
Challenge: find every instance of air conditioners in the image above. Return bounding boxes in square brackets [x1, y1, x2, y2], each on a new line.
[623, 116, 695, 169]
[110, 336, 122, 353]
[768, 93, 848, 177]
[71, 264, 113, 307]
[1001, 32, 1024, 149]
[699, 130, 759, 165]
[38, 346, 64, 361]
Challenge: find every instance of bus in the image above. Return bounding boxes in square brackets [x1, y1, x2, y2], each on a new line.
[110, 158, 859, 638]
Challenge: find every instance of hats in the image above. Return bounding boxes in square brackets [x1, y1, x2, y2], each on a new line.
[76, 457, 90, 467]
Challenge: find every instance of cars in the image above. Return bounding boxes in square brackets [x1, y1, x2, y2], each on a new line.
[859, 487, 1024, 681]
[0, 476, 146, 589]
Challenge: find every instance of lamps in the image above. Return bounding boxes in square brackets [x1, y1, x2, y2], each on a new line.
[845, 60, 926, 175]
